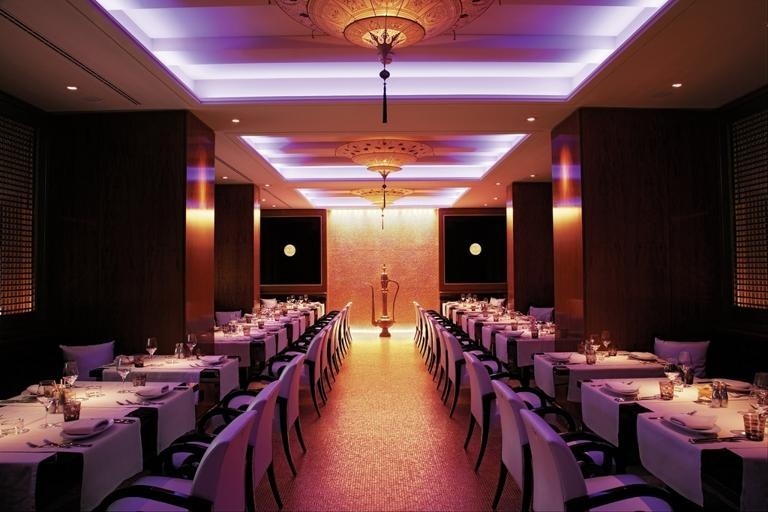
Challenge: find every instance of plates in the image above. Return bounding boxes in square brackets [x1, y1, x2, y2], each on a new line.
[22, 390, 38, 398]
[135, 390, 171, 399]
[60, 418, 115, 439]
[605, 384, 639, 395]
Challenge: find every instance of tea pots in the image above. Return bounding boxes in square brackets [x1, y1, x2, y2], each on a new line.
[367, 263, 399, 337]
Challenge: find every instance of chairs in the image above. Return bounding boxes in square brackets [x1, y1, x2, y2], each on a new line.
[491, 378, 626, 511]
[59, 340, 115, 382]
[212, 353, 307, 476]
[410, 299, 503, 418]
[519, 409, 681, 512]
[462, 350, 547, 472]
[654, 337, 710, 379]
[164, 379, 283, 512]
[97, 409, 258, 511]
[260, 301, 353, 418]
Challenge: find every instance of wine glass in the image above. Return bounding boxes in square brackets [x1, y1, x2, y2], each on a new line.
[116, 356, 130, 394]
[174, 333, 197, 361]
[37, 362, 81, 429]
[545, 330, 658, 364]
[458, 293, 554, 340]
[223, 293, 312, 337]
[146, 338, 158, 363]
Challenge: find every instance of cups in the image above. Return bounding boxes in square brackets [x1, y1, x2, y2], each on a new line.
[657, 350, 768, 442]
[134, 355, 145, 367]
[132, 373, 146, 387]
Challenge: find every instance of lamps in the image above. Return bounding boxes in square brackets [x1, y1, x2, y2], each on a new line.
[273, 1, 498, 124]
[350, 187, 414, 232]
[335, 139, 435, 209]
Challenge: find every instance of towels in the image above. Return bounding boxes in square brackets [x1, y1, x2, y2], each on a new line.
[543, 351, 572, 359]
[135, 384, 170, 397]
[714, 378, 751, 389]
[27, 385, 45, 396]
[630, 351, 656, 359]
[605, 380, 640, 392]
[64, 418, 109, 435]
[115, 354, 134, 363]
[670, 413, 718, 430]
